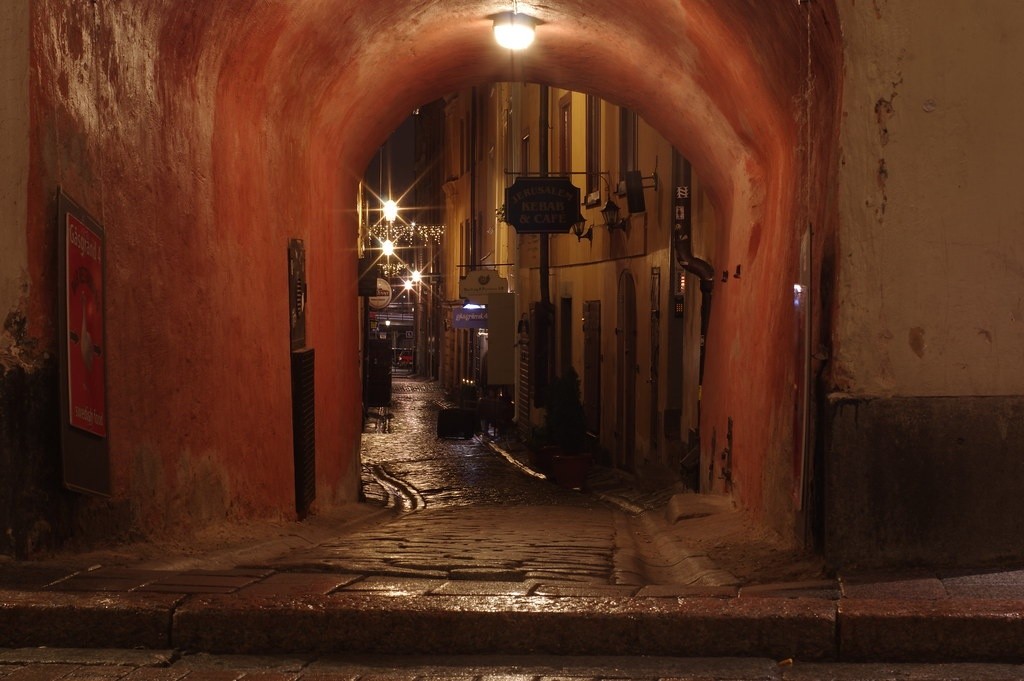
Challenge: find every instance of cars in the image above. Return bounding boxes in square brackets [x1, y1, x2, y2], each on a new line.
[398, 350, 413, 365]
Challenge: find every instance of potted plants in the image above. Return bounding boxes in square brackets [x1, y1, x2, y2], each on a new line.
[542, 376, 592, 482]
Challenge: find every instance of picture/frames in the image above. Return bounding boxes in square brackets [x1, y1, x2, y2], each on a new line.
[65, 213, 108, 437]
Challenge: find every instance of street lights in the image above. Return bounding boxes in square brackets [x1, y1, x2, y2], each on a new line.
[382, 199, 397, 238]
[383, 239, 394, 322]
[412, 270, 421, 303]
[404, 279, 412, 314]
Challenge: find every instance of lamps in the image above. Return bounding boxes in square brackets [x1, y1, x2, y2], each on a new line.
[572, 214, 595, 243]
[598, 200, 629, 232]
[487, 12, 547, 52]
